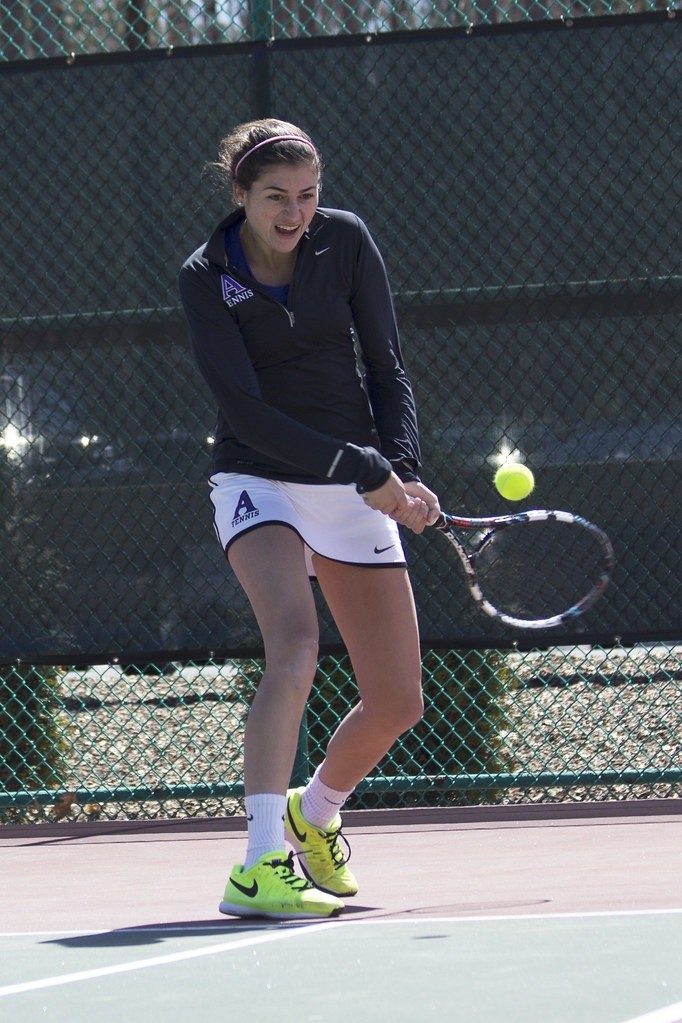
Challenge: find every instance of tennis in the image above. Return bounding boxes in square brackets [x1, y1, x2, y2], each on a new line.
[494, 463, 535, 501]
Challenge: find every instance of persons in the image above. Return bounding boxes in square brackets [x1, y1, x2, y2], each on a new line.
[178, 119, 441, 921]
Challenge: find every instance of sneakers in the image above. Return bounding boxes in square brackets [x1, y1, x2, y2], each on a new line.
[282, 786, 358, 898]
[219, 851, 345, 919]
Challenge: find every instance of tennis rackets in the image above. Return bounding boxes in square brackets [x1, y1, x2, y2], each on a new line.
[358, 482, 614, 631]
